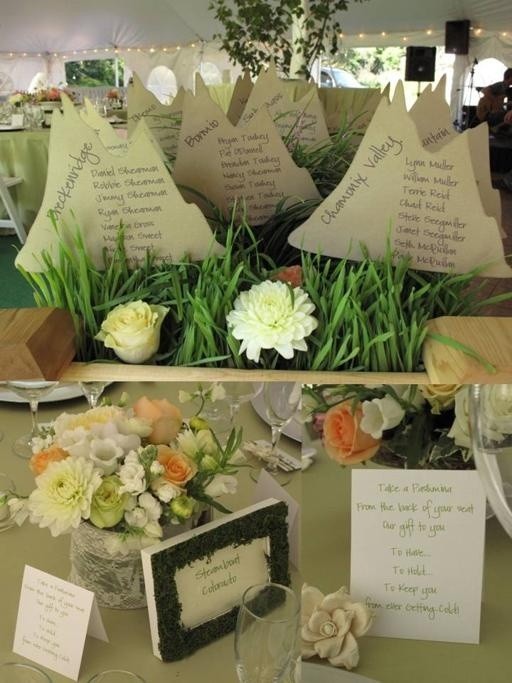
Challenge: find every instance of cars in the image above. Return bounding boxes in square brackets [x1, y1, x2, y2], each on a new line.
[318, 66, 366, 91]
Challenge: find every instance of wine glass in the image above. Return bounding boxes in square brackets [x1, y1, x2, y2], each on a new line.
[216, 383, 302, 487]
[5, 380, 111, 460]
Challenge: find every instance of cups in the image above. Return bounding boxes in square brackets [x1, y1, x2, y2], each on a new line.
[233, 581, 301, 682]
[470, 384, 511, 541]
[1, 662, 144, 683]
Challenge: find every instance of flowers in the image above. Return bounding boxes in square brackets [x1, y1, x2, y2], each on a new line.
[297, 384, 512, 471]
[34, 86, 75, 103]
[13, 212, 511, 372]
[8, 381, 254, 549]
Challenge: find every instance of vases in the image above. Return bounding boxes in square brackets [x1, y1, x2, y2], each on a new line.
[64, 513, 197, 610]
[39, 101, 62, 127]
